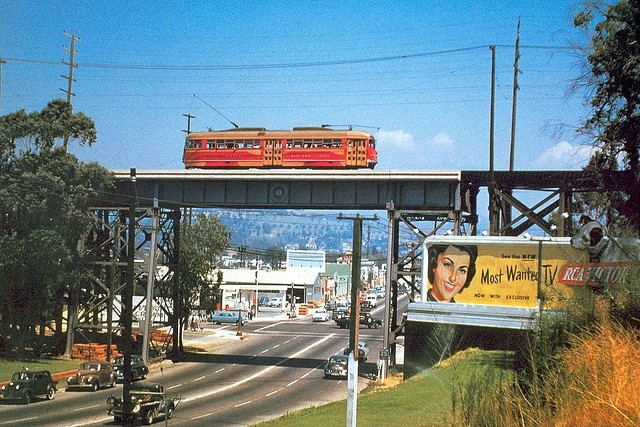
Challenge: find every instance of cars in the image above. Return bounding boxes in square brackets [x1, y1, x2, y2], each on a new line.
[330, 282, 417, 311]
[312, 307, 329, 321]
[209, 310, 247, 325]
[334, 311, 382, 327]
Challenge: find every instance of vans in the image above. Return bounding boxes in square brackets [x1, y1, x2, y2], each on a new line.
[24, 380, 26, 380]
[324, 342, 369, 379]
[67, 355, 148, 390]
[0, 371, 57, 405]
[107, 382, 176, 426]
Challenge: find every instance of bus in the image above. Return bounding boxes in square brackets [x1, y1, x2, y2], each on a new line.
[182, 126, 378, 168]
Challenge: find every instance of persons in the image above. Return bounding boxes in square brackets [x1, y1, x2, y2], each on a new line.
[427, 245, 478, 303]
